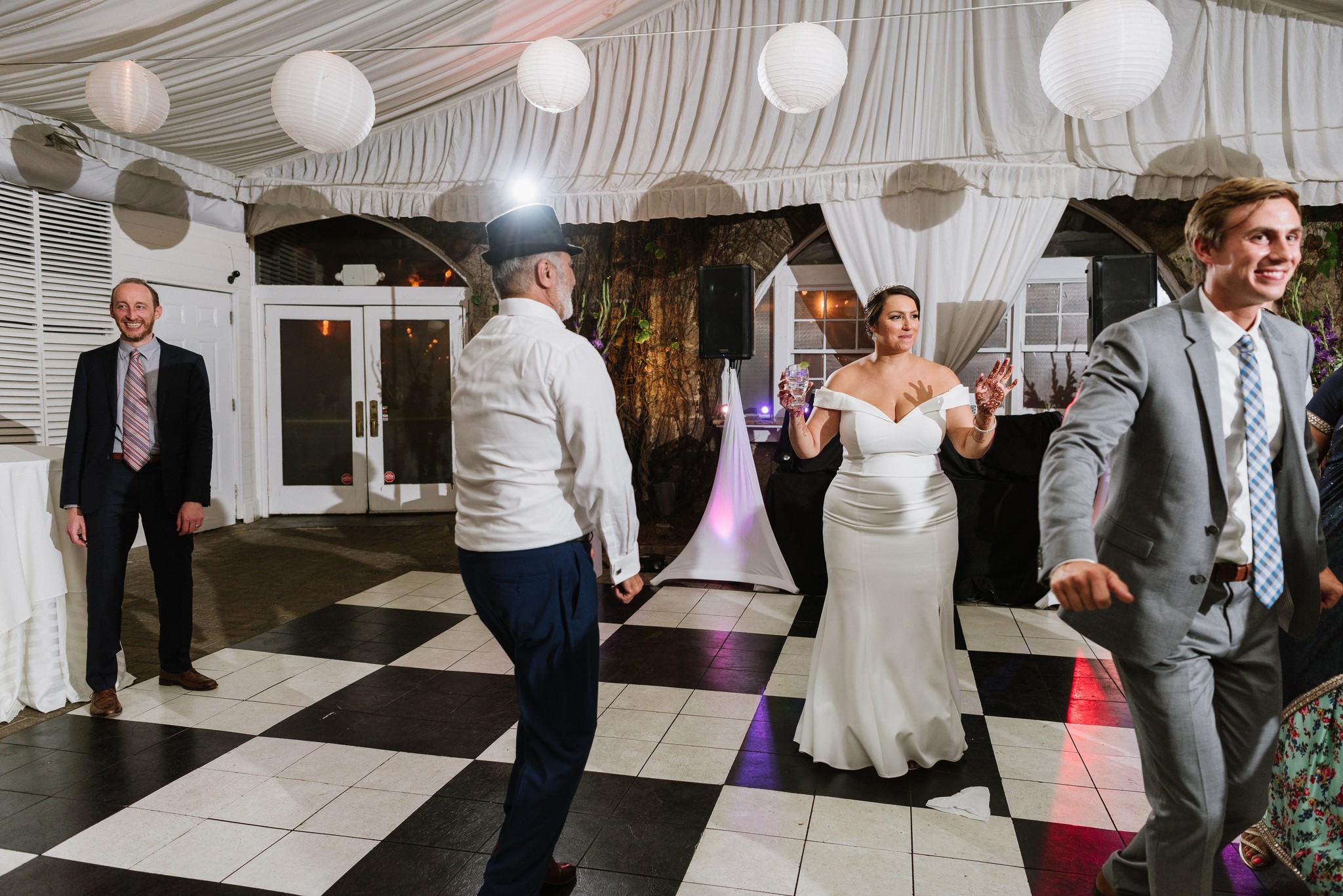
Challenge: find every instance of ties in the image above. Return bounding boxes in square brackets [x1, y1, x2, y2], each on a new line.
[1235, 333, 1286, 608]
[123, 350, 152, 470]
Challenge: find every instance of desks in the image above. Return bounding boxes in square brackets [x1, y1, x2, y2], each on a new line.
[1, 441, 137, 728]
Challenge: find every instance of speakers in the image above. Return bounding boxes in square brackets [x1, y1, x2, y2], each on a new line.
[698, 263, 756, 360]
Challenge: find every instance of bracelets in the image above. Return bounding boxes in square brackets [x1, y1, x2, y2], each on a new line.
[973, 414, 997, 433]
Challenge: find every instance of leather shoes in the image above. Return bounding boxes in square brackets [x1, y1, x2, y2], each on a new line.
[90, 685, 123, 718]
[478, 841, 577, 895]
[159, 669, 219, 691]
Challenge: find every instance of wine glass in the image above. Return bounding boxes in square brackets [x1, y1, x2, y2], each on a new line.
[785, 364, 809, 409]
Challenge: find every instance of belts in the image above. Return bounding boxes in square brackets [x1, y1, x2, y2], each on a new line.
[1211, 563, 1250, 582]
[112, 453, 159, 463]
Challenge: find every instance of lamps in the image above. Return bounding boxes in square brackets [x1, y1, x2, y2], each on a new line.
[85, 60, 170, 136]
[270, 50, 377, 154]
[517, 35, 591, 113]
[756, 21, 849, 116]
[1037, 0, 1174, 124]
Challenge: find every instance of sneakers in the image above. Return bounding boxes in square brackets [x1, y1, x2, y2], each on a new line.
[1089, 869, 1117, 895]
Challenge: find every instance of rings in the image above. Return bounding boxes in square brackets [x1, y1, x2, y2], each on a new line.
[195, 525, 200, 528]
[1000, 381, 1007, 385]
[777, 393, 782, 397]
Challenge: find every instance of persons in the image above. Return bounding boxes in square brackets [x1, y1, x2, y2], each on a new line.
[59, 278, 220, 717]
[449, 203, 645, 896]
[777, 285, 1020, 782]
[1037, 173, 1342, 896]
[1283, 365, 1342, 701]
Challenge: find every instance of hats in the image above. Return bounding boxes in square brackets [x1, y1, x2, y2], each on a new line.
[479, 205, 583, 263]
